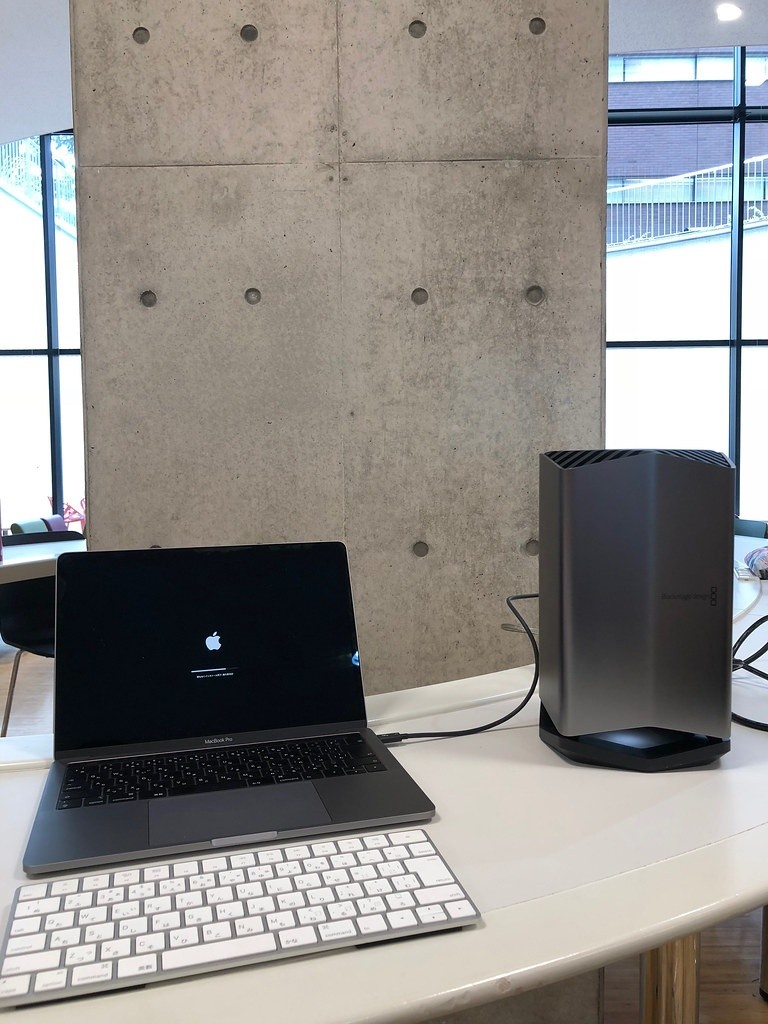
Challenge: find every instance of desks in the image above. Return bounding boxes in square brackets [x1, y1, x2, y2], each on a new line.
[0, 538, 87, 583]
[0, 534, 768, 1024]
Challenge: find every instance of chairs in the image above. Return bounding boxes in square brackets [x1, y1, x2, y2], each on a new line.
[734, 519, 768, 538]
[0, 530, 85, 738]
[11, 519, 48, 535]
[40, 513, 67, 531]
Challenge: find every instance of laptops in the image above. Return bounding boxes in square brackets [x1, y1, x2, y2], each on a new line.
[21, 541, 436, 873]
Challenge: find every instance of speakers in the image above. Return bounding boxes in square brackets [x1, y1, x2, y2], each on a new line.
[539, 450, 736, 773]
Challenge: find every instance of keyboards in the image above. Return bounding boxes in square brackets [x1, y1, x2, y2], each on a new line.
[0, 828, 481, 1011]
